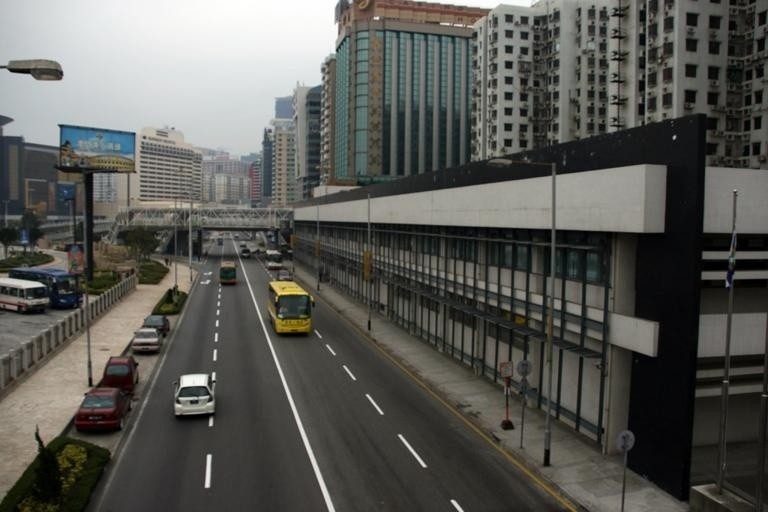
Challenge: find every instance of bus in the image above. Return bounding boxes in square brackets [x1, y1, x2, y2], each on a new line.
[266, 280, 316, 336]
[219, 260, 237, 285]
[0, 277, 51, 314]
[8, 266, 84, 311]
[264, 249, 283, 270]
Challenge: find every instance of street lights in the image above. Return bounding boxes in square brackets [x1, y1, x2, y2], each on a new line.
[485, 156, 561, 467]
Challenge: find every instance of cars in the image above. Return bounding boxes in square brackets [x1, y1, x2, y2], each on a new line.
[171, 371, 218, 417]
[102, 354, 140, 390]
[142, 312, 170, 334]
[217, 232, 251, 258]
[276, 269, 293, 281]
[75, 385, 131, 433]
[132, 326, 164, 352]
